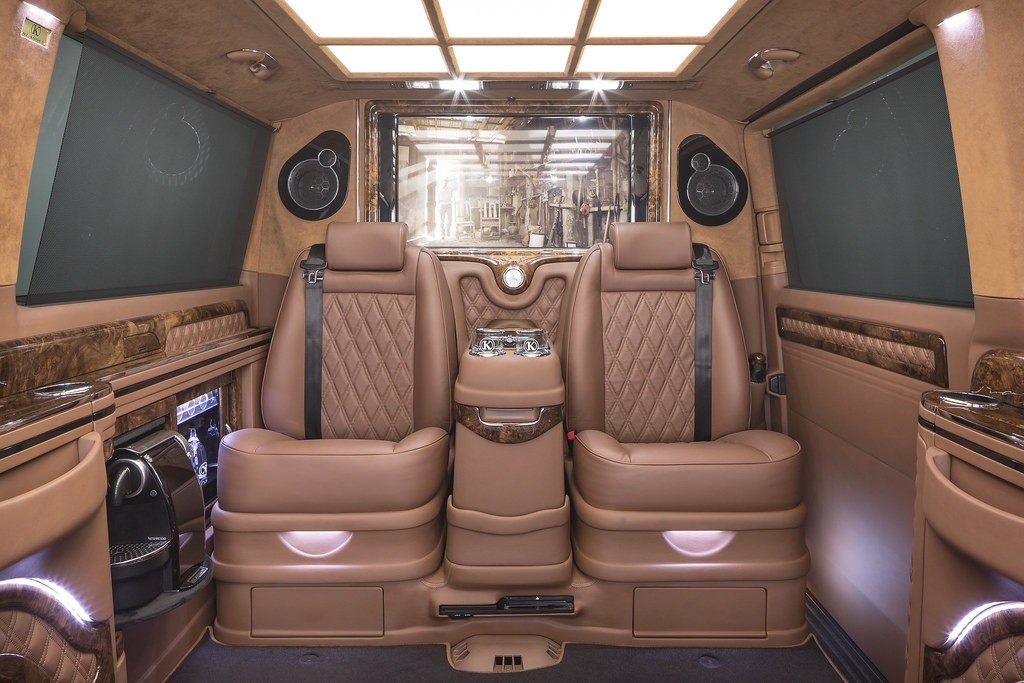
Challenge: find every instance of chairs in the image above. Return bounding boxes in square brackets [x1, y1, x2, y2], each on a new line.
[209, 222, 459, 646]
[563, 222, 809, 648]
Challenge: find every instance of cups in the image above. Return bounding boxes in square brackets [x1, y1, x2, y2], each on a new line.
[516, 330, 548, 357]
[472, 327, 503, 355]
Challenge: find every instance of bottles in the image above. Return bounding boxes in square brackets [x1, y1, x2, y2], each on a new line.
[188, 428, 207, 485]
[207, 419, 219, 467]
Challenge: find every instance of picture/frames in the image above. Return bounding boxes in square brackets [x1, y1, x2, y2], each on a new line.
[377, 113, 651, 252]
